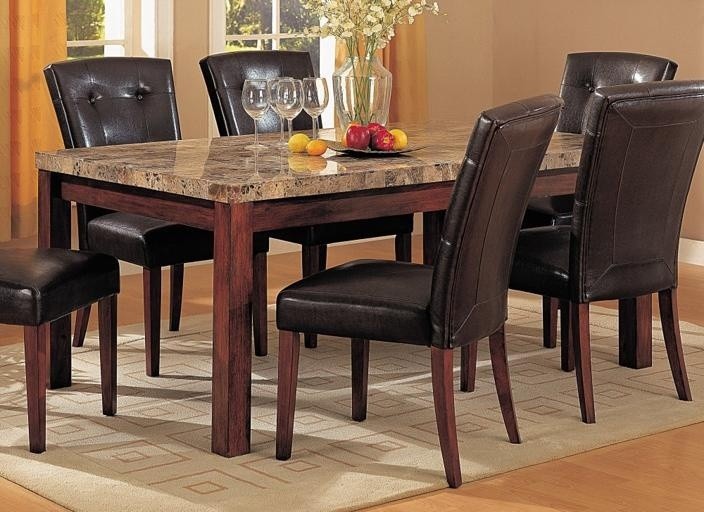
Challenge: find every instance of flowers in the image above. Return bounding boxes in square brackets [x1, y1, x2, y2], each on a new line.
[298, 0, 447, 125]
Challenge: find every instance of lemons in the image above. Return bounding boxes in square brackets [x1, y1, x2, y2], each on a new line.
[288, 133, 310, 153]
[305, 139, 328, 156]
[390, 128, 408, 150]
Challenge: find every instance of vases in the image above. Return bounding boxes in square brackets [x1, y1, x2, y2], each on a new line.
[330, 55, 393, 135]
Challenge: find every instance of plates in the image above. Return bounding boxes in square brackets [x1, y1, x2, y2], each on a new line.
[317, 138, 428, 155]
[326, 155, 429, 172]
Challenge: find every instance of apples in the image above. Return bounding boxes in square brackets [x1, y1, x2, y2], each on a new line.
[343, 123, 395, 150]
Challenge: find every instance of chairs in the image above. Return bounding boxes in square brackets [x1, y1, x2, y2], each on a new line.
[458, 79, 704, 425]
[520, 49, 678, 349]
[274, 92, 568, 489]
[41, 54, 270, 378]
[198, 47, 414, 360]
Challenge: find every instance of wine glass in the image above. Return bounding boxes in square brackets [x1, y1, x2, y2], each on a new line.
[240, 75, 330, 152]
[248, 154, 295, 182]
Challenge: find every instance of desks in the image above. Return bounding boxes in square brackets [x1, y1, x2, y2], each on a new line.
[31, 126, 583, 460]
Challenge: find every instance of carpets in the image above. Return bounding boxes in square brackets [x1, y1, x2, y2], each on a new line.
[0, 287, 703, 512]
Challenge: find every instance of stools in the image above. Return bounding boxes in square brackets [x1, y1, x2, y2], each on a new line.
[0, 243, 120, 454]
